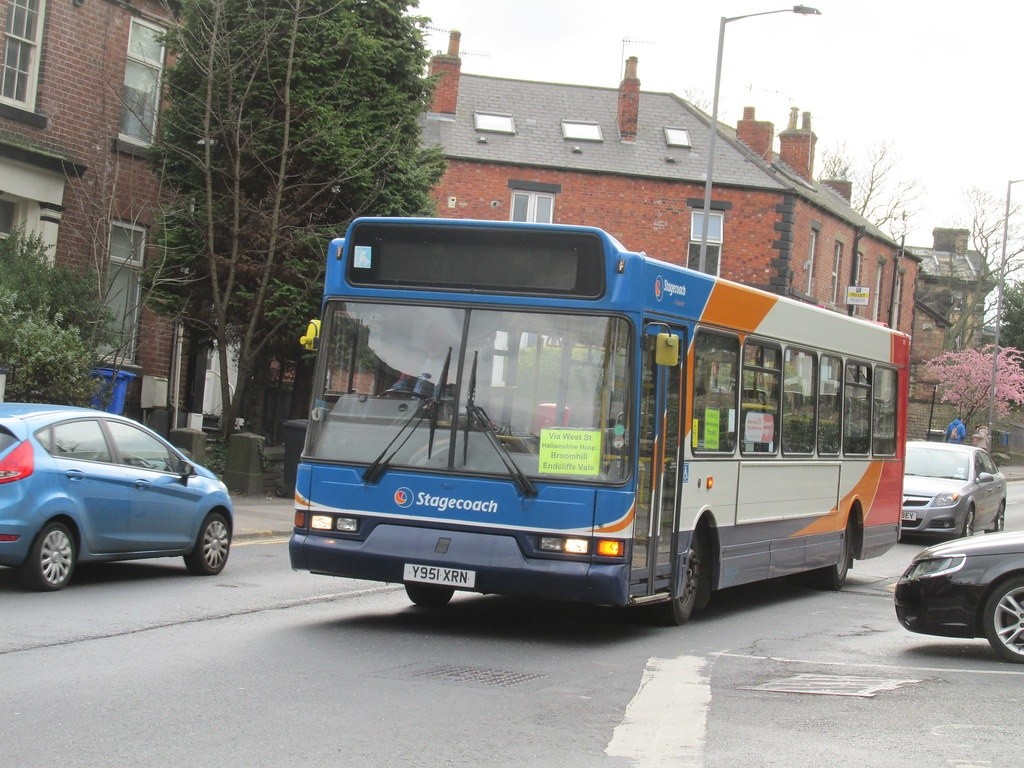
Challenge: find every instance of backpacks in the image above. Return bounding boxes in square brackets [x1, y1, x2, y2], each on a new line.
[950, 424, 962, 439]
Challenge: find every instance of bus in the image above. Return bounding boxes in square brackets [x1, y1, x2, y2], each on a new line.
[288, 214, 912, 628]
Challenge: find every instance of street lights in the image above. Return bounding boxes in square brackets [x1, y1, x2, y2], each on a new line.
[699, 3, 823, 275]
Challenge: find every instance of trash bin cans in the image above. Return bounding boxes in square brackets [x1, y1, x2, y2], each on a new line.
[279, 417, 308, 497]
[925, 429, 947, 443]
[86, 367, 137, 416]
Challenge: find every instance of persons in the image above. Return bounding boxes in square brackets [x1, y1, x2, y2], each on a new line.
[971, 426, 989, 450]
[946, 416, 965, 444]
[391, 323, 467, 419]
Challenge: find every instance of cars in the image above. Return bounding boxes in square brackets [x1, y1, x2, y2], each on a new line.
[894, 529, 1024, 664]
[901, 440, 1008, 540]
[0, 400, 235, 592]
